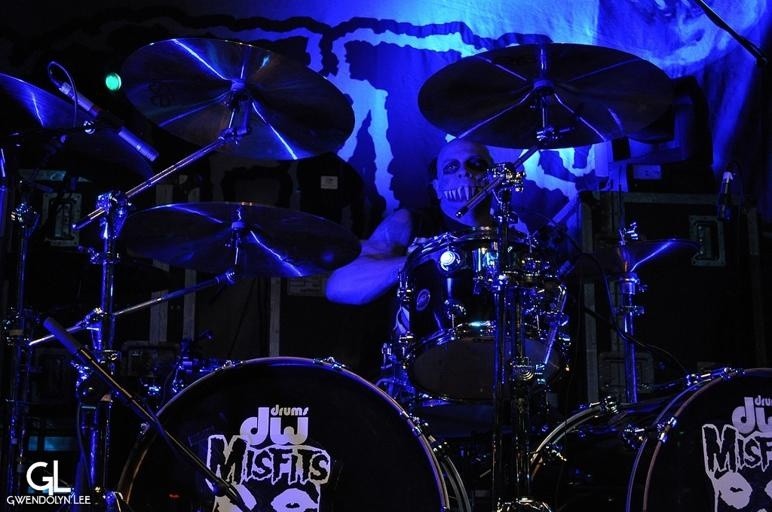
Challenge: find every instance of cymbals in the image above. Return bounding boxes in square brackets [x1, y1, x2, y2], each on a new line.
[124, 202, 363, 279]
[613, 236, 699, 266]
[418, 42, 676, 149]
[128, 37, 354, 159]
[0, 75, 76, 128]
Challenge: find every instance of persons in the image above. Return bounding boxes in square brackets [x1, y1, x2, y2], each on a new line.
[322, 136, 531, 355]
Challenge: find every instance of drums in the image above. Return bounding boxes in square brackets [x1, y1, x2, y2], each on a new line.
[529, 366, 772, 510]
[117, 356, 471, 510]
[396, 230, 571, 403]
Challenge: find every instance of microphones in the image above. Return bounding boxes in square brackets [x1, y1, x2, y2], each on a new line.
[58, 82, 161, 163]
[716, 176, 729, 221]
